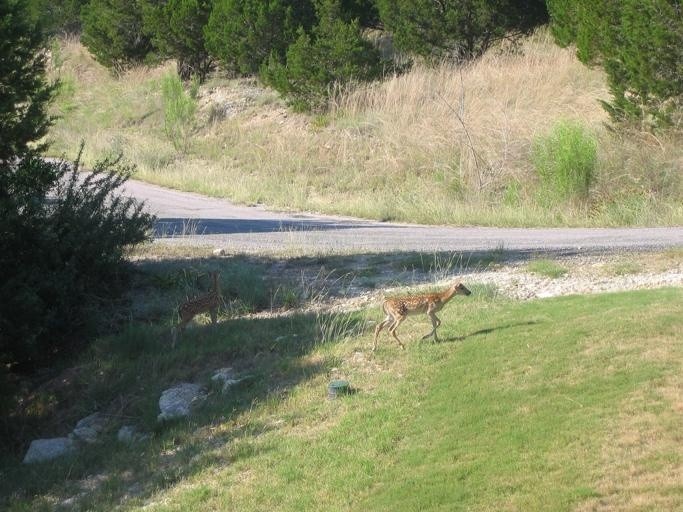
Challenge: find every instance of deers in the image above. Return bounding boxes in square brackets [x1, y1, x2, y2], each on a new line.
[169, 268, 222, 350]
[372, 277, 472, 352]
